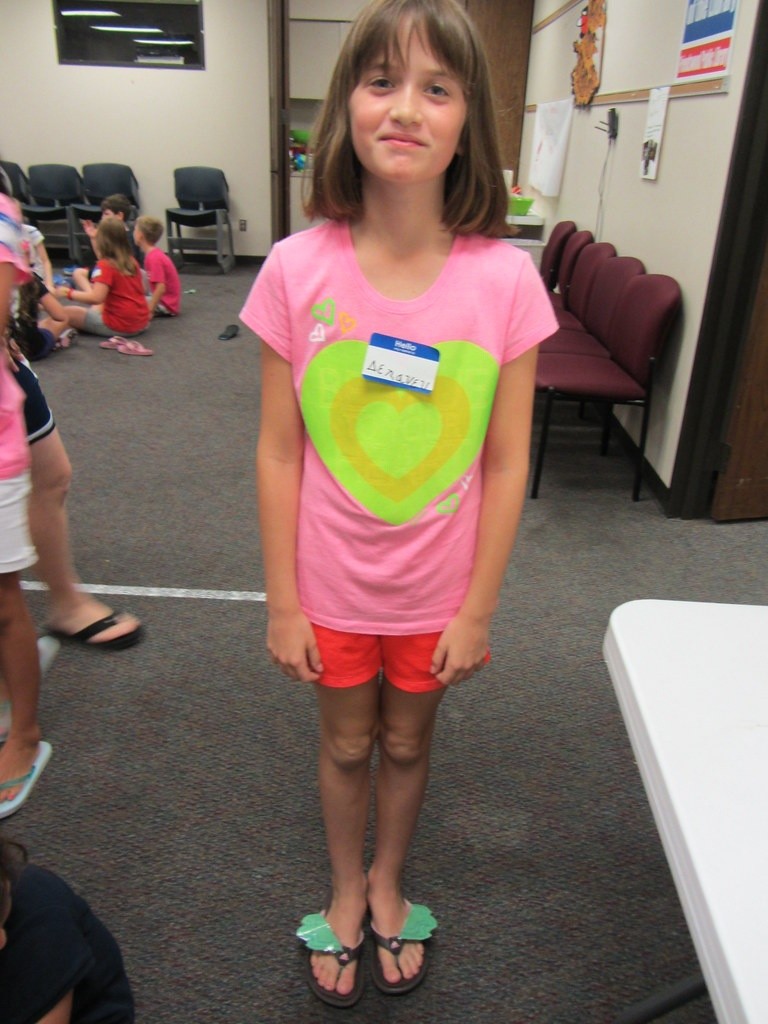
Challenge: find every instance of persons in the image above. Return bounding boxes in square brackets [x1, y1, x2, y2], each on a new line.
[0, 194, 145, 824]
[54, 215, 152, 339]
[132, 215, 182, 320]
[235, 0, 558, 1007]
[72, 193, 146, 291]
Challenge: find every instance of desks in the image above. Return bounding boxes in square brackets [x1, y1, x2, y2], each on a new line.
[604, 601, 768, 1024]
[502, 206, 546, 226]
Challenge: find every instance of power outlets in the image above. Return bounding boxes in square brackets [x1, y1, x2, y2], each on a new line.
[239, 219, 247, 232]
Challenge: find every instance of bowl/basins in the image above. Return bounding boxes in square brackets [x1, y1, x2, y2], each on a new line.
[507, 197, 534, 216]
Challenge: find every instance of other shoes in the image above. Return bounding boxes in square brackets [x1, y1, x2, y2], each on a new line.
[53, 275, 66, 285]
[63, 264, 79, 276]
[52, 328, 79, 351]
[218, 325, 239, 340]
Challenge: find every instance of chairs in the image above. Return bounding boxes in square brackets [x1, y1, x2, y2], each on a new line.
[529, 254, 646, 425]
[164, 166, 238, 274]
[0, 160, 29, 206]
[537, 243, 615, 333]
[538, 220, 576, 281]
[21, 163, 81, 266]
[69, 163, 141, 268]
[528, 274, 683, 502]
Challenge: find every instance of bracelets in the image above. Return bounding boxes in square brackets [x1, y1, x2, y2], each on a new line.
[67, 288, 73, 300]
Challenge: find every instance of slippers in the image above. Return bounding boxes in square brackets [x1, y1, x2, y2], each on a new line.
[0, 741, 51, 820]
[100, 335, 128, 349]
[118, 341, 153, 356]
[295, 908, 369, 1009]
[370, 897, 438, 997]
[1, 637, 60, 739]
[46, 607, 142, 648]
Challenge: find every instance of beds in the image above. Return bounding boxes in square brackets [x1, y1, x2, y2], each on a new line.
[537, 230, 592, 308]
